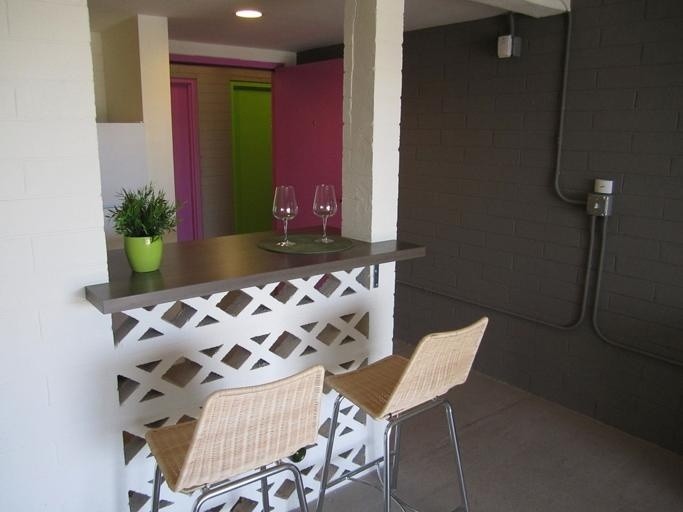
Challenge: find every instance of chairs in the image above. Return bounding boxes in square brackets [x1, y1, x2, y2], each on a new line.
[315, 316, 489, 511]
[144, 364, 325, 512]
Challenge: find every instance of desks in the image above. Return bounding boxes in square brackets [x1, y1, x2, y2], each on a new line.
[80, 220, 432, 512]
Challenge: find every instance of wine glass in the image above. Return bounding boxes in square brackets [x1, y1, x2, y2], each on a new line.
[271, 185, 299, 248]
[312, 183, 337, 244]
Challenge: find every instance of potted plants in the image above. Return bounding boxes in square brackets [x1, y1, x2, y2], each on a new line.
[103, 180, 188, 273]
